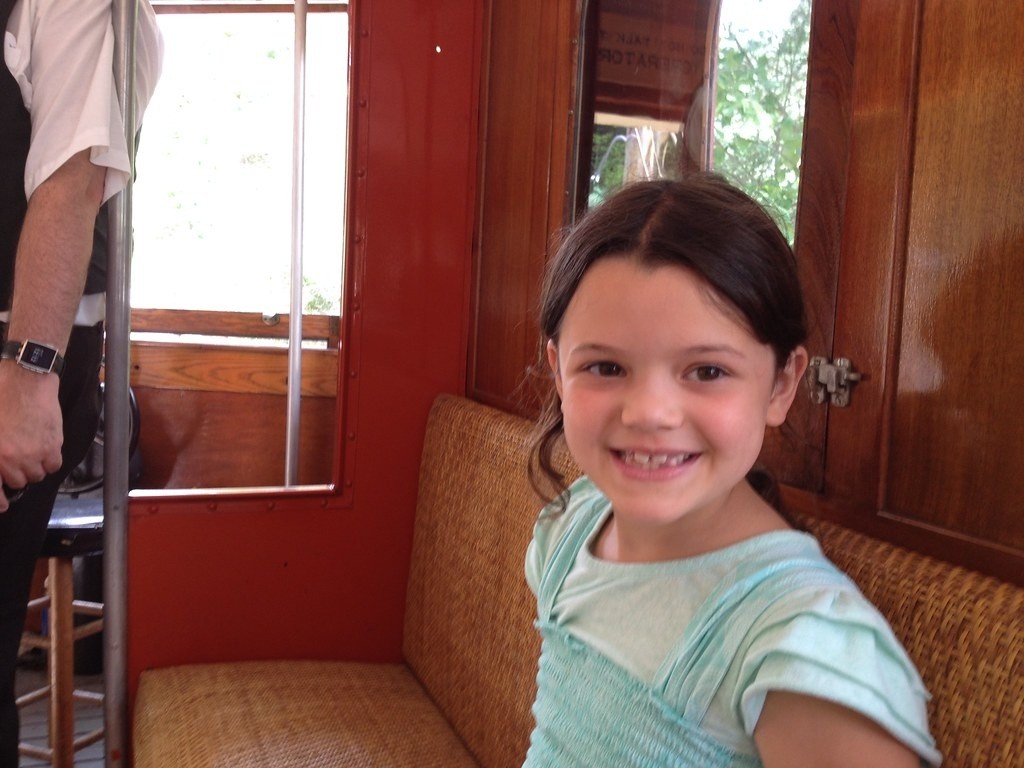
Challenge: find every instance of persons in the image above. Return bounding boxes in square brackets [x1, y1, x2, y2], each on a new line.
[0, 1, 164, 768]
[522, 178, 944, 767]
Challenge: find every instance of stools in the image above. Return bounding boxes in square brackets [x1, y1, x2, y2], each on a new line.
[17, 496, 108, 768]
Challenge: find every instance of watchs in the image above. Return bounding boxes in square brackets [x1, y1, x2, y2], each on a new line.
[0, 341, 67, 377]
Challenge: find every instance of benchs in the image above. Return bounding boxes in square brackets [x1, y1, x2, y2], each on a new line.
[130, 392, 1024, 768]
